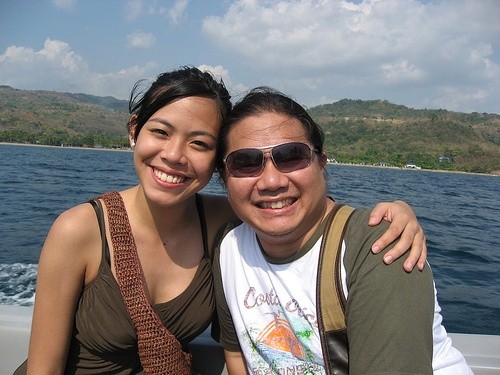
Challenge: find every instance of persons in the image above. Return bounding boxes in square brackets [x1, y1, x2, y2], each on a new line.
[210, 86, 474, 375]
[14, 64, 427, 375]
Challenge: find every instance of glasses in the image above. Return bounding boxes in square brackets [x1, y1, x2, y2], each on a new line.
[223, 141, 318, 179]
